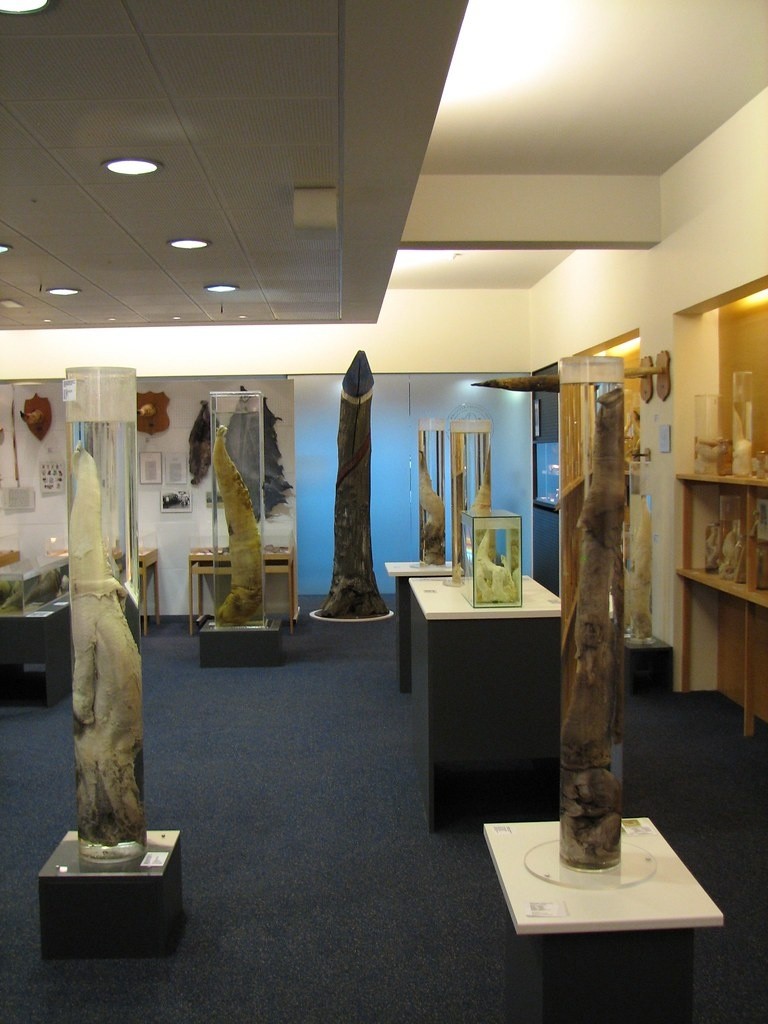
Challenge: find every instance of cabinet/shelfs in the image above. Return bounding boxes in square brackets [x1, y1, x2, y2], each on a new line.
[677, 473, 768, 736]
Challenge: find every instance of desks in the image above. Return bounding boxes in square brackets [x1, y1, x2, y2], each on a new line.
[136, 547, 160, 636]
[188, 548, 300, 634]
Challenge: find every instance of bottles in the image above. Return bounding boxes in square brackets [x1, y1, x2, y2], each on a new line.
[716, 439, 734, 475]
[754, 450, 768, 480]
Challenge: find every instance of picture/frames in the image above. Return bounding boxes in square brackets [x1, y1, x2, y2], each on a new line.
[160, 488, 192, 512]
